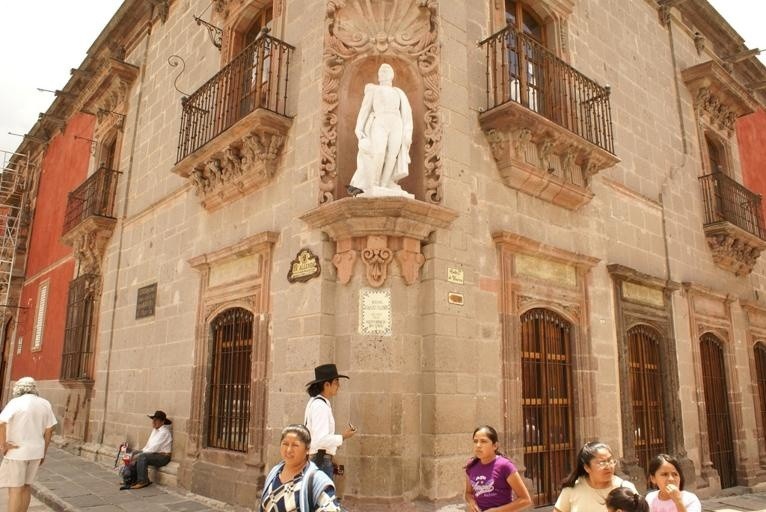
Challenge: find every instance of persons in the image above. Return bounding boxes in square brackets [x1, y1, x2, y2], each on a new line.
[601, 487, 650, 512]
[463, 424, 533, 512]
[551, 441, 640, 512]
[119, 410, 172, 488]
[0, 376, 58, 511]
[644, 452, 701, 512]
[260, 424, 340, 512]
[302, 363, 357, 486]
[350, 63, 413, 197]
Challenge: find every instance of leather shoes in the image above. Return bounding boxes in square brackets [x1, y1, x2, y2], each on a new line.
[130, 480, 150, 489]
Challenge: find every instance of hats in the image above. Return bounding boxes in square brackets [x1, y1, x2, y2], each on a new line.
[306, 364, 349, 387]
[148, 411, 171, 424]
